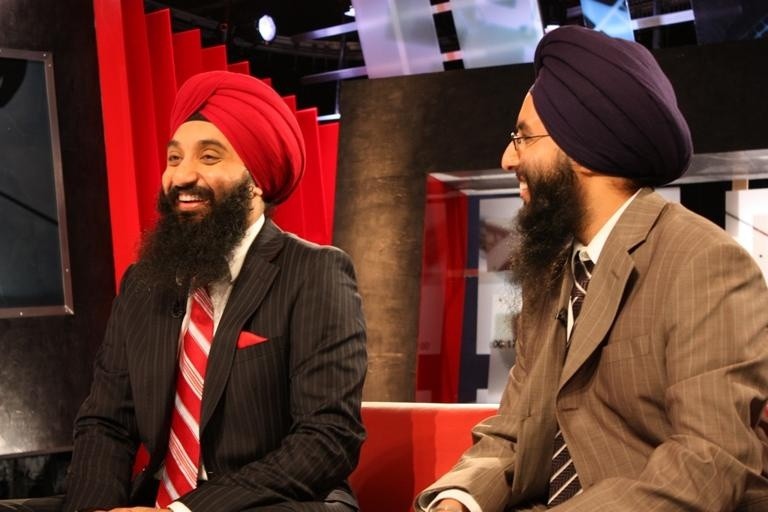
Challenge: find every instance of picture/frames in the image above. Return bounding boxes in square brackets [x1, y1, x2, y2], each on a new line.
[415, 148, 768, 407]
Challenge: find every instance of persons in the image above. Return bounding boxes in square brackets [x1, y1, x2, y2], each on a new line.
[410, 25, 768, 511]
[62, 69, 369, 512]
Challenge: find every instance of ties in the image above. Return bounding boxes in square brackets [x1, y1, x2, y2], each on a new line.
[548, 251, 595, 509]
[153, 287, 214, 509]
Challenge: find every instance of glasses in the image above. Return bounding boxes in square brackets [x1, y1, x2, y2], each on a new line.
[511, 132, 550, 150]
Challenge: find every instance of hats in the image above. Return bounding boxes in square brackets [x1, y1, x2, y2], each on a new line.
[172, 70, 306, 205]
[532, 24, 693, 186]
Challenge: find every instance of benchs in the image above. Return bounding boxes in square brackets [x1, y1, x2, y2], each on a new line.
[339, 401, 500, 512]
